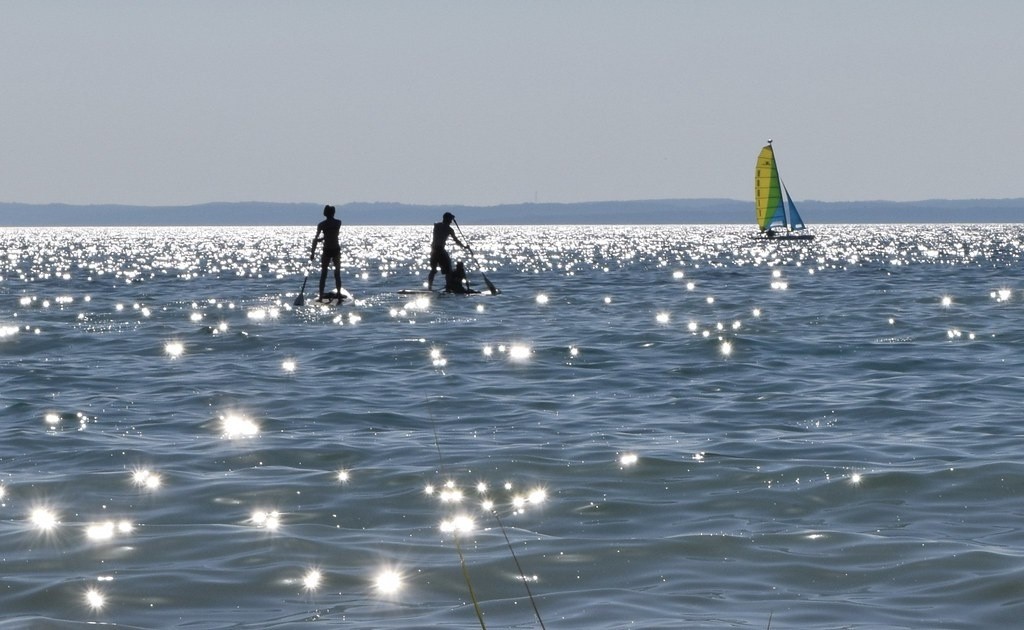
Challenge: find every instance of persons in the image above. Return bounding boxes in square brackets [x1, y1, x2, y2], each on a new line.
[428, 212, 470, 292]
[310, 205, 343, 303]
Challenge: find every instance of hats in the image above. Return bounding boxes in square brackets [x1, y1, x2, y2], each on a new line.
[323, 205, 335, 218]
[443, 212, 455, 220]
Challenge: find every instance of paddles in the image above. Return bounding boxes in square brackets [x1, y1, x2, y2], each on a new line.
[450, 214, 497, 296]
[294, 235, 319, 308]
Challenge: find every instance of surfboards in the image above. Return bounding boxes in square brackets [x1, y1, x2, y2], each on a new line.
[396, 287, 502, 297]
[308, 286, 355, 309]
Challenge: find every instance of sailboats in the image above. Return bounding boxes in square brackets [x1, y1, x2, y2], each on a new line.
[751, 139, 817, 239]
